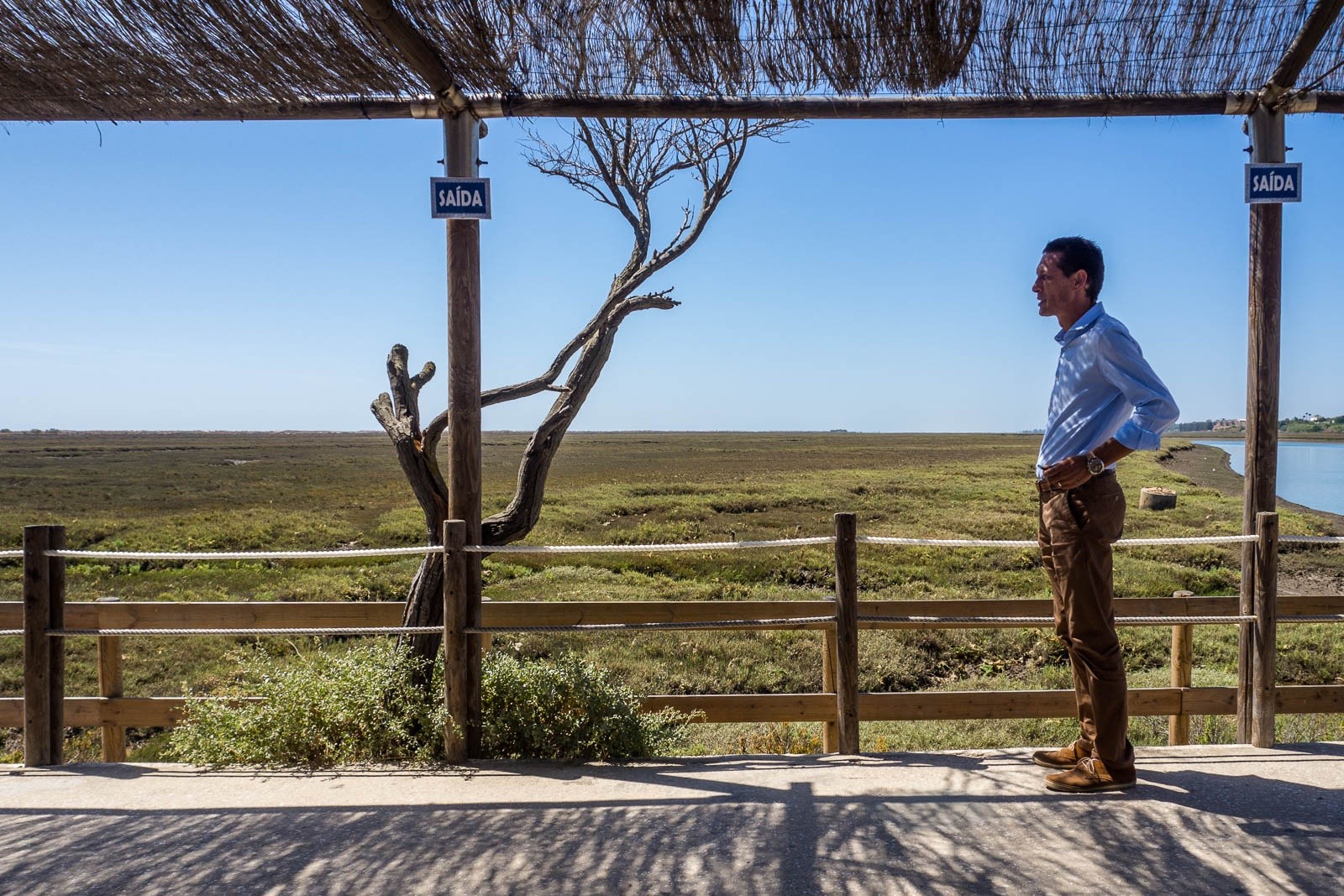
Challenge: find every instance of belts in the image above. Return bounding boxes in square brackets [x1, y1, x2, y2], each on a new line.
[1034, 480, 1051, 493]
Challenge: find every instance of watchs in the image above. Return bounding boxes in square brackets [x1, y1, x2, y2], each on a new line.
[1085, 450, 1105, 475]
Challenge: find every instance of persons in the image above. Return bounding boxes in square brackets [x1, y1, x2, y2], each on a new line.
[1031, 236, 1181, 792]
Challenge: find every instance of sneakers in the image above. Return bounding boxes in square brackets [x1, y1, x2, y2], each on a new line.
[1044, 757, 1137, 791]
[1033, 740, 1090, 768]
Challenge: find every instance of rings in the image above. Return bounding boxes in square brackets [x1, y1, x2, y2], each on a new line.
[1058, 482, 1062, 486]
